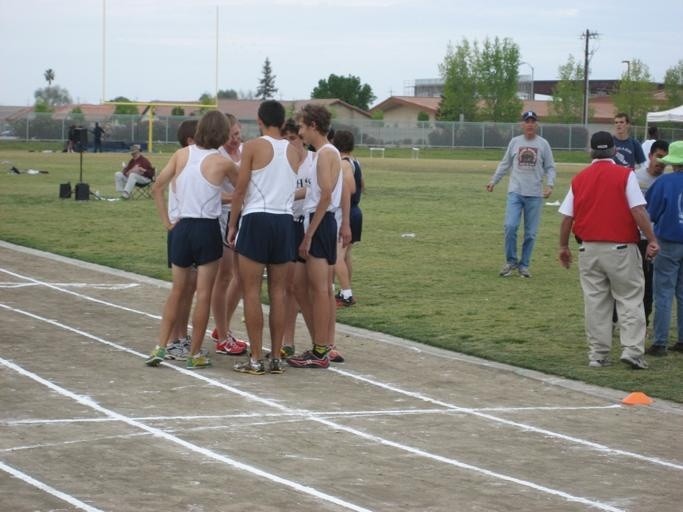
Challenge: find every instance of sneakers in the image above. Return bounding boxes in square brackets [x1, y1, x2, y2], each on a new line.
[336, 292, 356, 308]
[589, 341, 683, 370]
[500, 263, 518, 277]
[117, 191, 128, 200]
[518, 264, 532, 277]
[144, 328, 344, 375]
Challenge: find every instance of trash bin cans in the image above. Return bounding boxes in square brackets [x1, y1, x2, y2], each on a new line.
[412, 148, 419, 160]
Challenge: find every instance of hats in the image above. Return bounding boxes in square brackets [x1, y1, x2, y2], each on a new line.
[590, 131, 613, 150]
[655, 140, 683, 166]
[129, 144, 141, 154]
[523, 112, 537, 121]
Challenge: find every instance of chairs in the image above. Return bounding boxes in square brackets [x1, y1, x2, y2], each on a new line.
[126, 166, 156, 201]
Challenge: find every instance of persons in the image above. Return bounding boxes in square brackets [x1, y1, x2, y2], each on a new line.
[332, 130, 365, 308]
[114, 144, 153, 199]
[143, 100, 352, 374]
[66, 122, 104, 153]
[486, 111, 556, 278]
[557, 132, 659, 369]
[612, 113, 682, 356]
